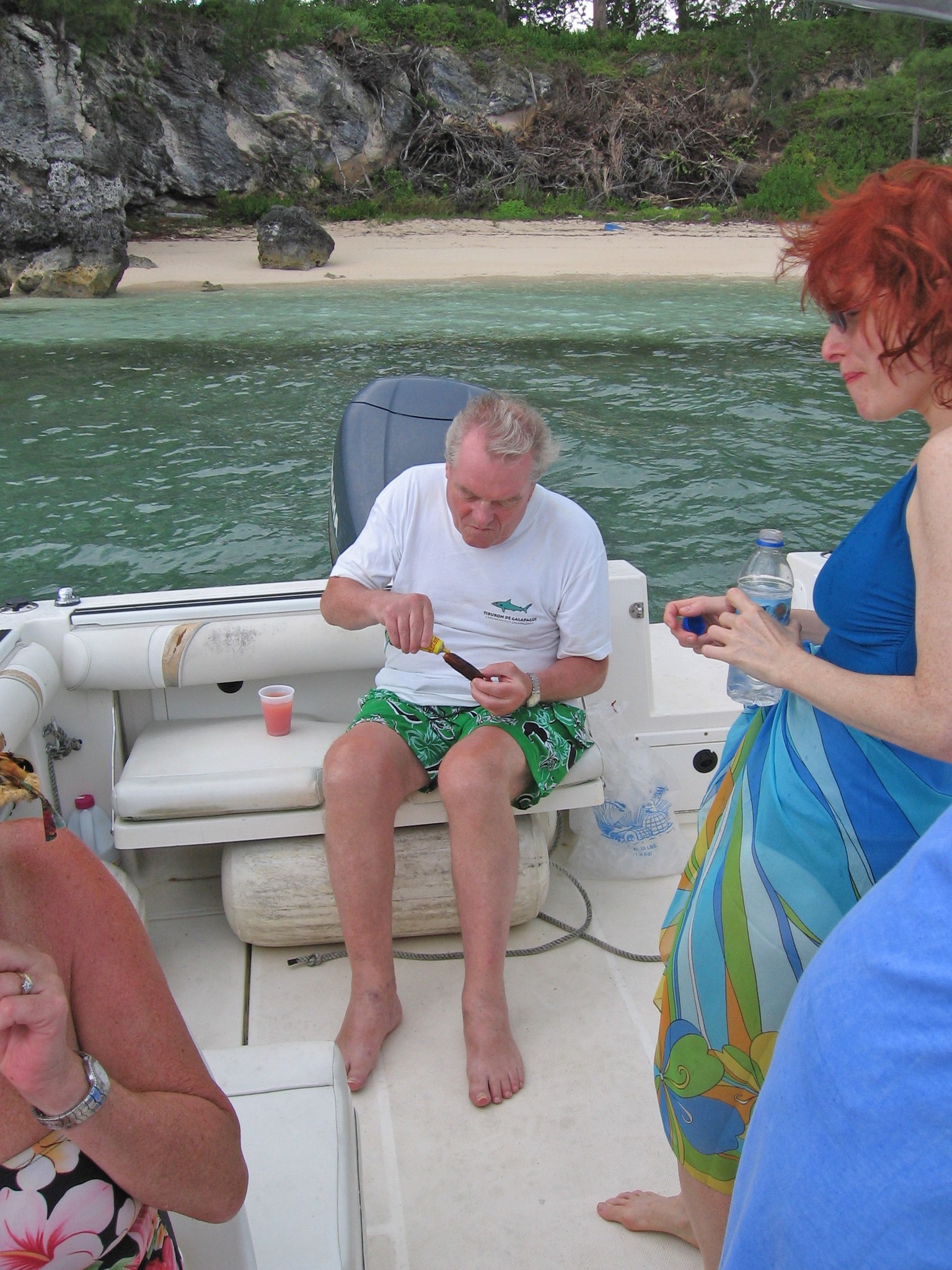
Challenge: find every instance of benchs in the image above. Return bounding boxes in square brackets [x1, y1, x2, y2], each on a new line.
[60, 611, 606, 850]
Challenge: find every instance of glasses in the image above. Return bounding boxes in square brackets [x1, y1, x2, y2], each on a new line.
[829, 275, 939, 334]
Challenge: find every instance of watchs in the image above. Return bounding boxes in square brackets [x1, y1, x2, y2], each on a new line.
[524, 672, 541, 707]
[31, 1049, 111, 1130]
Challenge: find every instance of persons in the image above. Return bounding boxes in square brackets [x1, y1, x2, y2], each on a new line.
[0, 815, 249, 1270]
[594, 162, 950, 1270]
[320, 396, 614, 1106]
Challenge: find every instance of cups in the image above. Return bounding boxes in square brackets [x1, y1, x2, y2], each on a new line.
[257, 683, 295, 736]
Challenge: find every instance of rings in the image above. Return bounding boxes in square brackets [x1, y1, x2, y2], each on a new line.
[14, 970, 35, 997]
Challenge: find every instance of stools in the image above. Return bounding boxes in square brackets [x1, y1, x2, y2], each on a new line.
[166, 1042, 369, 1270]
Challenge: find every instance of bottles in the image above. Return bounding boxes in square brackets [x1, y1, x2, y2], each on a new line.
[727, 529, 793, 705]
[64, 795, 132, 877]
